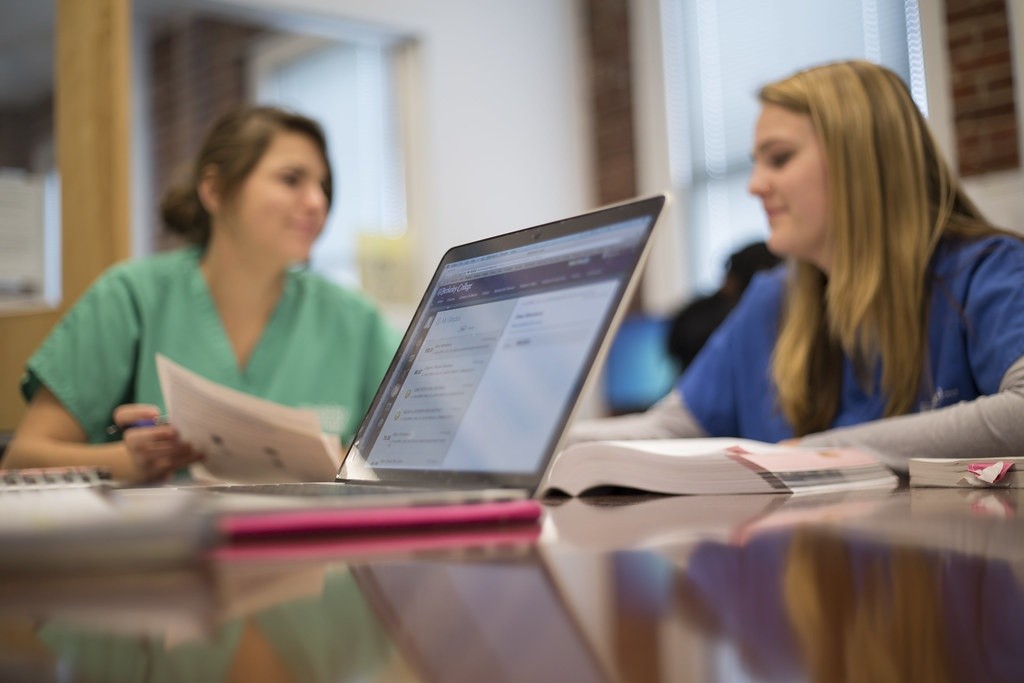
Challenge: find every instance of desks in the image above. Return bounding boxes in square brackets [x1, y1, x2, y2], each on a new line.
[0, 482, 1024, 683]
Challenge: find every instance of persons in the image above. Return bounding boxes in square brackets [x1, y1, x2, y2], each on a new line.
[0, 106, 405, 481]
[559, 60, 1023, 465]
[38, 567, 395, 682]
[679, 525, 1024, 682]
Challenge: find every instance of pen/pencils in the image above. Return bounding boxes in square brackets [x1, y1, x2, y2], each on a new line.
[217, 499, 542, 536]
[106, 415, 168, 437]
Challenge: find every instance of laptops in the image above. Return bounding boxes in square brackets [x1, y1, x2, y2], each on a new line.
[108, 188, 670, 532]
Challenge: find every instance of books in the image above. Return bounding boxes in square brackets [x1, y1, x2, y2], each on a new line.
[538, 435, 900, 497]
[908, 457, 1024, 489]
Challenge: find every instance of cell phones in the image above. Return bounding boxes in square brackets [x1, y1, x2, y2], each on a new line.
[213, 496, 544, 541]
